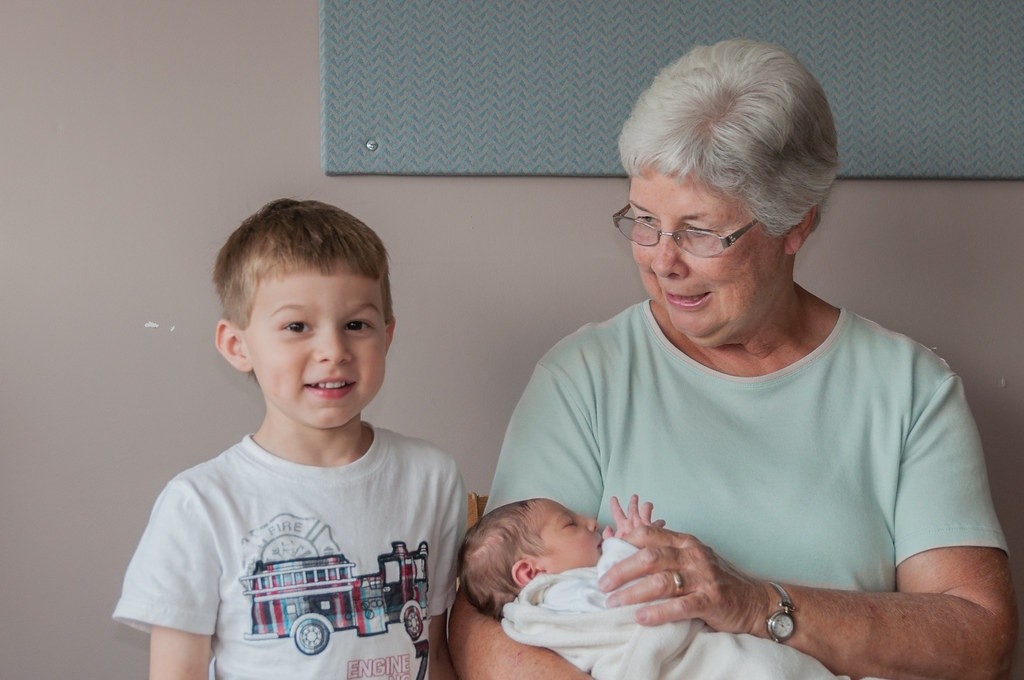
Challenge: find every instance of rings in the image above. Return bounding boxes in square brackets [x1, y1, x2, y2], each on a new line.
[671, 570, 684, 597]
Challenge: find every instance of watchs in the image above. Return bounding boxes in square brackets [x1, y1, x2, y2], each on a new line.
[765, 581, 797, 644]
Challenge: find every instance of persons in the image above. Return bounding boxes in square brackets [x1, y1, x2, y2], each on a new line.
[447, 39, 1024, 680]
[111, 198, 468, 680]
[459, 494, 886, 680]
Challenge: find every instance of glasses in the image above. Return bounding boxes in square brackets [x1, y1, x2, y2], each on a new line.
[611, 204, 759, 258]
